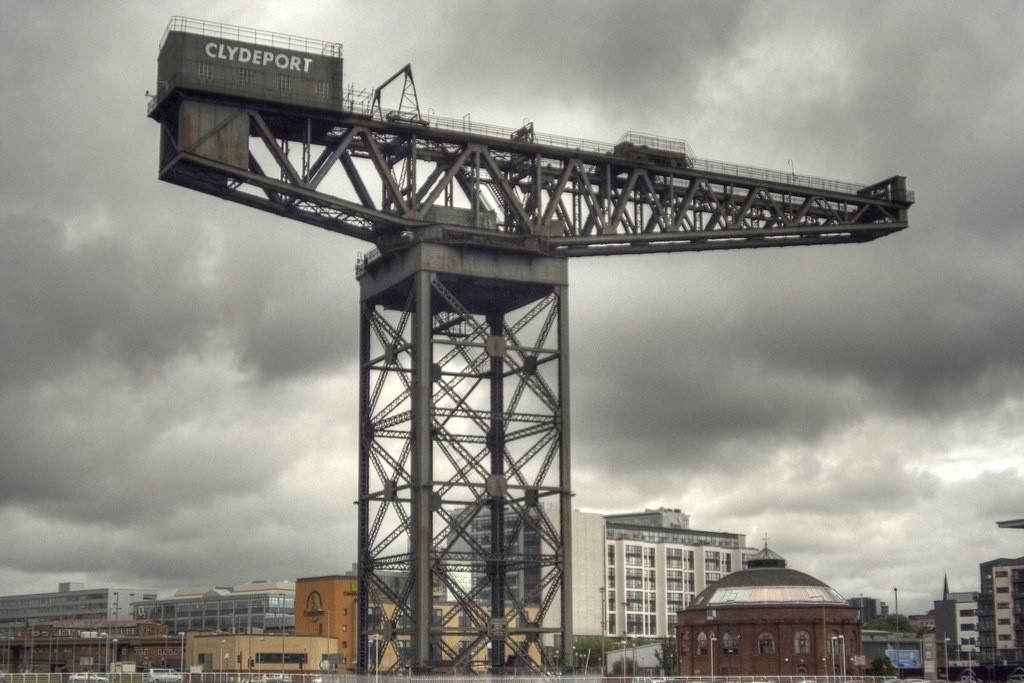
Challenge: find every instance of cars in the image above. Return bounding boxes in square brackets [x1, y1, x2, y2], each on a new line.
[242, 674, 293, 683]
[68, 672, 109, 683]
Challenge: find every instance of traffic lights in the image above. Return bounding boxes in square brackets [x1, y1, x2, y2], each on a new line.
[236, 654, 240, 663]
[299, 658, 303, 670]
[335, 664, 338, 669]
[250, 659, 255, 668]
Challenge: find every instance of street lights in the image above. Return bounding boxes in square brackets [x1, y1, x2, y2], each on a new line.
[838, 635, 847, 683]
[810, 595, 829, 677]
[101, 632, 108, 672]
[30, 619, 44, 673]
[710, 637, 717, 677]
[112, 638, 119, 662]
[621, 640, 627, 683]
[555, 651, 559, 673]
[157, 623, 168, 669]
[219, 638, 226, 681]
[893, 586, 900, 680]
[319, 608, 332, 675]
[944, 638, 952, 683]
[964, 636, 972, 683]
[831, 636, 838, 681]
[631, 643, 637, 680]
[573, 646, 587, 675]
[278, 593, 284, 683]
[177, 630, 185, 682]
[369, 633, 380, 683]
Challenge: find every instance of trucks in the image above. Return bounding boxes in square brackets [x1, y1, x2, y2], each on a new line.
[110, 661, 136, 674]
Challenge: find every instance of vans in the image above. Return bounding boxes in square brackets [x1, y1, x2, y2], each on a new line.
[143, 668, 183, 683]
